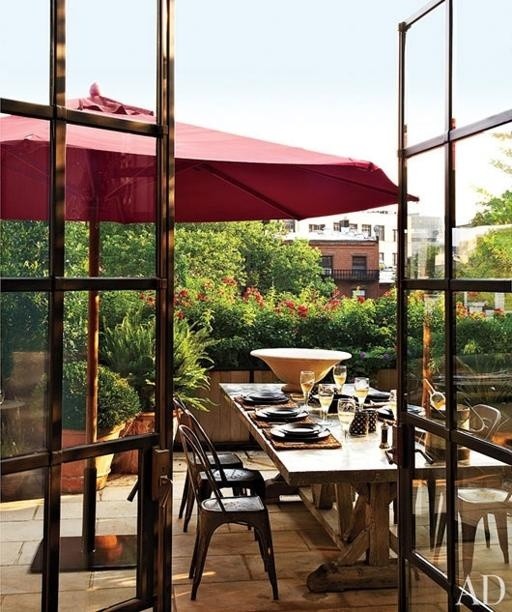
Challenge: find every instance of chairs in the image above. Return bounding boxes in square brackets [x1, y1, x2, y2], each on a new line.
[179, 382, 511, 601]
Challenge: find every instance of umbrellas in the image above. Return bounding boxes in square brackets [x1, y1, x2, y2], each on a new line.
[1, 83, 420, 551]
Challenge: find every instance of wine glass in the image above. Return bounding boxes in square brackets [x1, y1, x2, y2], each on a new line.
[300, 366, 369, 449]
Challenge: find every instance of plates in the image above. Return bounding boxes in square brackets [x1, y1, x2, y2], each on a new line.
[367, 392, 422, 419]
[240, 390, 331, 442]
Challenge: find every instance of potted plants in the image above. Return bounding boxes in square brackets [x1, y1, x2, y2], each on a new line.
[33, 309, 218, 493]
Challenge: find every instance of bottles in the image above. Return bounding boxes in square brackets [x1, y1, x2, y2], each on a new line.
[422, 379, 447, 412]
[379, 420, 389, 449]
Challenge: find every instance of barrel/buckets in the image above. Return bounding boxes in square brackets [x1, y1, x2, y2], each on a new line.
[426, 404, 485, 460]
